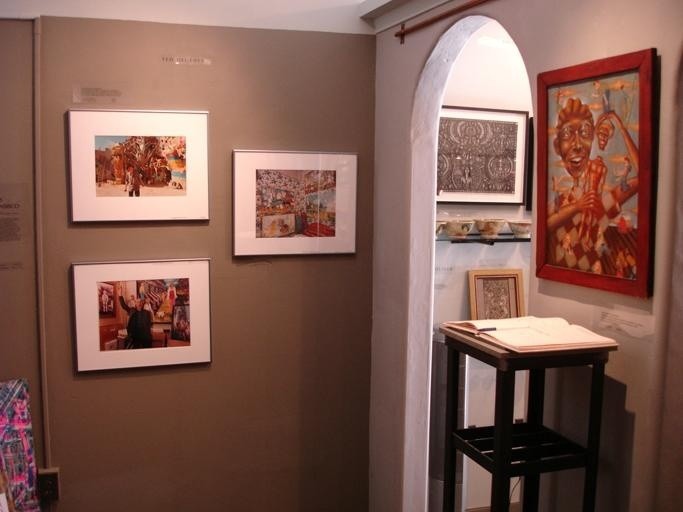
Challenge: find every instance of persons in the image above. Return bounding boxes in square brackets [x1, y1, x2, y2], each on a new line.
[124, 164, 145, 197]
[101, 289, 109, 312]
[118, 281, 188, 348]
[547, 98, 638, 280]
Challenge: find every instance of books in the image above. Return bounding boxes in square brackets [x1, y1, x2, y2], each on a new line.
[443, 314, 619, 354]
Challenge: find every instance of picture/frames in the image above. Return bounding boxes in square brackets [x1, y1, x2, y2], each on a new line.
[436, 105, 530, 205]
[231, 149, 361, 257]
[68, 108, 212, 372]
[537, 47, 657, 301]
[468, 269, 526, 320]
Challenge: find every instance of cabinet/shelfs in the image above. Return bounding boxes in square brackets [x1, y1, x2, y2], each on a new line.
[439, 325, 618, 511]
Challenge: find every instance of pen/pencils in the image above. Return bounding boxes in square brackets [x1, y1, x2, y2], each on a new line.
[476, 325, 530, 332]
